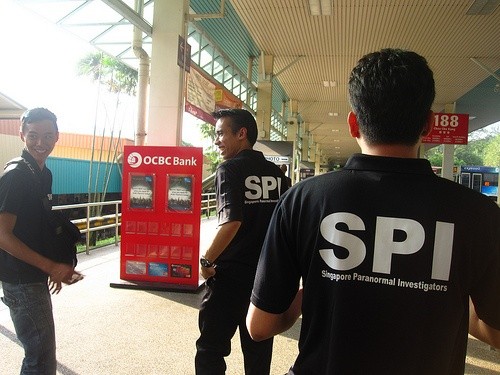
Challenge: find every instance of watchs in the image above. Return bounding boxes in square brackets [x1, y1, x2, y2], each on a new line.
[200, 256, 214, 268]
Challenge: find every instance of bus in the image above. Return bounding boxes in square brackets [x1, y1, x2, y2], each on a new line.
[456, 165, 498, 200]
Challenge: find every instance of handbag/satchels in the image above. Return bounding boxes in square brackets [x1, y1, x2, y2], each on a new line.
[4, 159, 81, 269]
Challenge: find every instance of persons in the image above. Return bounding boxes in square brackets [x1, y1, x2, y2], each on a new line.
[0, 108, 84, 375]
[246, 48, 500, 375]
[195, 109, 290, 375]
